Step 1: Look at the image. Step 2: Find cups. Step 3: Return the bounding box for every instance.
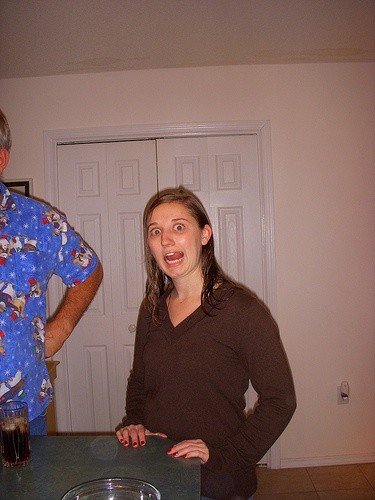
[0,401,32,468]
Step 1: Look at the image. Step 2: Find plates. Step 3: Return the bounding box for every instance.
[60,478,161,500]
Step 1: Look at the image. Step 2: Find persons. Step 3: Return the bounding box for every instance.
[0,110,103,435]
[115,187,297,500]
